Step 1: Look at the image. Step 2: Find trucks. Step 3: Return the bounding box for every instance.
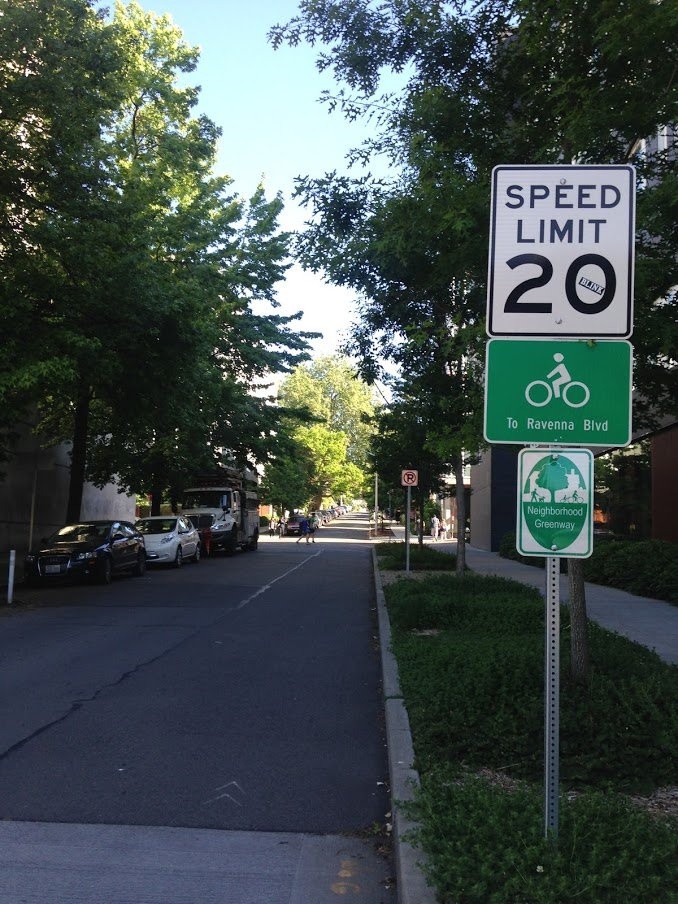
[179,475,260,556]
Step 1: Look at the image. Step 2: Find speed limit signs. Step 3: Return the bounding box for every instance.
[485,164,636,339]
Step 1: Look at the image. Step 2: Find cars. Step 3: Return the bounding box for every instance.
[135,514,202,568]
[285,504,354,536]
[25,519,146,586]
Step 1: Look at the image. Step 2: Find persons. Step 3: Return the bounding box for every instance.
[395,509,401,525]
[309,513,316,544]
[296,515,310,545]
[268,516,277,536]
[439,518,447,541]
[277,516,285,538]
[431,514,439,542]
